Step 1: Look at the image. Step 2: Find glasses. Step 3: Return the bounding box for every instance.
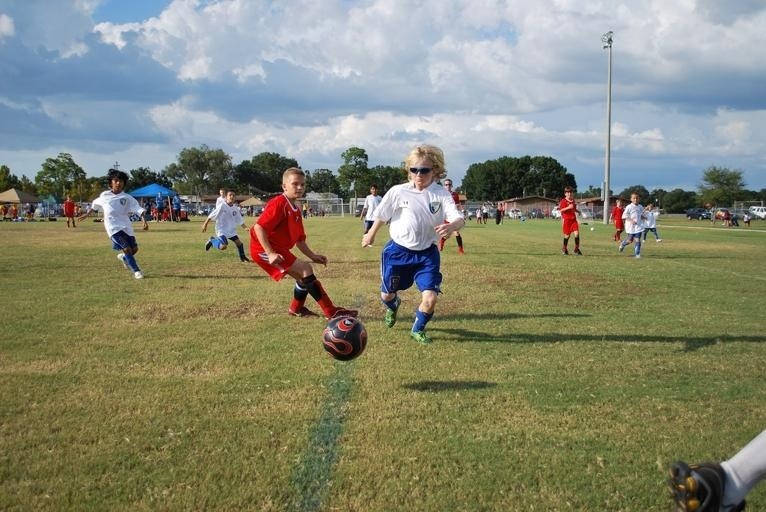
[409,167,434,174]
[444,183,452,186]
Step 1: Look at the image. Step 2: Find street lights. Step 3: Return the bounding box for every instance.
[600,31,615,225]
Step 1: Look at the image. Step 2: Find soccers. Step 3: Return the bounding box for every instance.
[322,317,368,362]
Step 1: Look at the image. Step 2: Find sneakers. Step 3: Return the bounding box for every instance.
[411,328,433,344]
[619,242,624,253]
[117,252,127,271]
[135,272,145,281]
[204,239,213,251]
[67,225,69,227]
[288,307,319,320]
[241,257,255,265]
[459,248,464,254]
[73,225,76,227]
[575,249,583,257]
[614,234,616,241]
[325,308,358,322]
[656,239,662,243]
[643,239,646,244]
[617,240,622,244]
[562,247,569,255]
[636,254,640,259]
[384,298,402,329]
[439,240,443,251]
[671,459,747,512]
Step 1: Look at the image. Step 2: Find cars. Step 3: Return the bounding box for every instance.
[528,208,544,218]
[687,208,711,220]
[356,205,364,216]
[715,208,732,220]
[729,210,756,223]
[509,209,522,218]
[469,207,477,216]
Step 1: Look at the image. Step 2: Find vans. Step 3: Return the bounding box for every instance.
[552,206,595,219]
[749,206,766,220]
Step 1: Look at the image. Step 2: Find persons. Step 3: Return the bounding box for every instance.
[156,192,164,223]
[144,198,158,221]
[64,195,77,228]
[463,200,551,225]
[202,190,253,263]
[78,169,149,280]
[189,207,215,216]
[643,204,662,243]
[74,202,91,218]
[172,193,182,222]
[609,199,626,242]
[237,206,267,217]
[0,202,63,224]
[438,178,464,255]
[710,208,754,229]
[667,426,765,512]
[560,186,583,256]
[216,188,226,209]
[363,145,465,343]
[360,184,383,235]
[302,201,331,219]
[619,192,649,258]
[249,168,359,322]
